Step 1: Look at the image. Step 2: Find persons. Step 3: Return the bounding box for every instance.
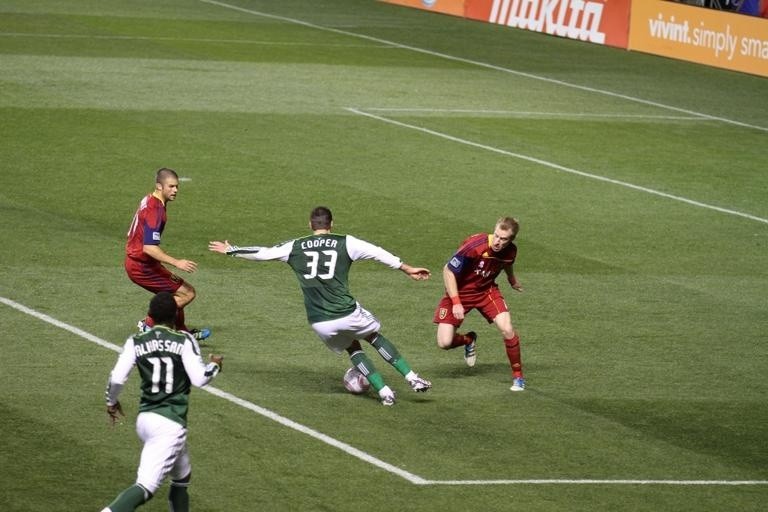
[100,292,223,512]
[433,216,528,391]
[124,167,210,340]
[208,205,431,406]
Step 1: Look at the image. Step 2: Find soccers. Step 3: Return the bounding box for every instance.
[342,365,370,393]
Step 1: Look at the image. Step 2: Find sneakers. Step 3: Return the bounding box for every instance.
[190,329,210,339]
[510,378,524,391]
[382,394,395,406]
[409,378,432,392]
[463,332,476,366]
[138,319,150,333]
